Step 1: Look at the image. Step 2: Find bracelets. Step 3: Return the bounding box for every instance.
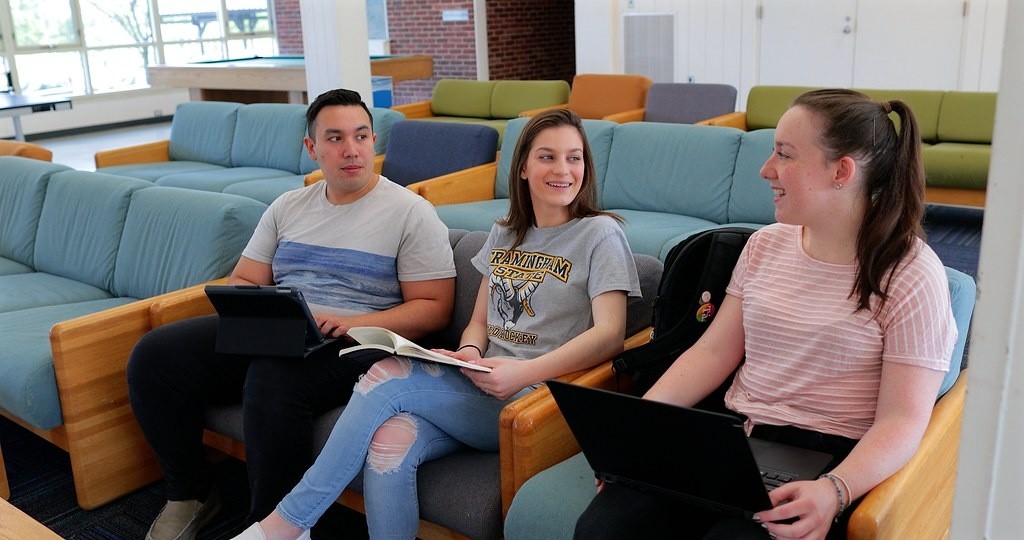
[457,344,481,356]
[819,472,852,523]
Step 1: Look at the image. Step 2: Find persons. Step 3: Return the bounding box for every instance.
[229,109,644,540]
[572,88,958,540]
[127,88,456,540]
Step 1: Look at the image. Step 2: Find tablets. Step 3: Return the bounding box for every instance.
[205,284,324,344]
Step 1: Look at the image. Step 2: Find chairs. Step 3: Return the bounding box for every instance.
[519,73,652,123]
[604,82,737,125]
[305,120,499,194]
[512,264,968,540]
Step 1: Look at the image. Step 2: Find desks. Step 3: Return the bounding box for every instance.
[146,54,432,104]
[0,91,72,142]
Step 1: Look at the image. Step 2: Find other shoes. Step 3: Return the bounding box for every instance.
[229,520,312,540]
[145,485,221,540]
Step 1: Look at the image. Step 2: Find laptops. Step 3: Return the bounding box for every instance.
[542,378,839,520]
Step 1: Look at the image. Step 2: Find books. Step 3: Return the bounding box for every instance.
[338,326,492,374]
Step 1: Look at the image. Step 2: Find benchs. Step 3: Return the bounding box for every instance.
[418,117,782,265]
[716,85,997,209]
[148,219,664,540]
[1,155,267,510]
[390,79,570,149]
[93,101,404,205]
